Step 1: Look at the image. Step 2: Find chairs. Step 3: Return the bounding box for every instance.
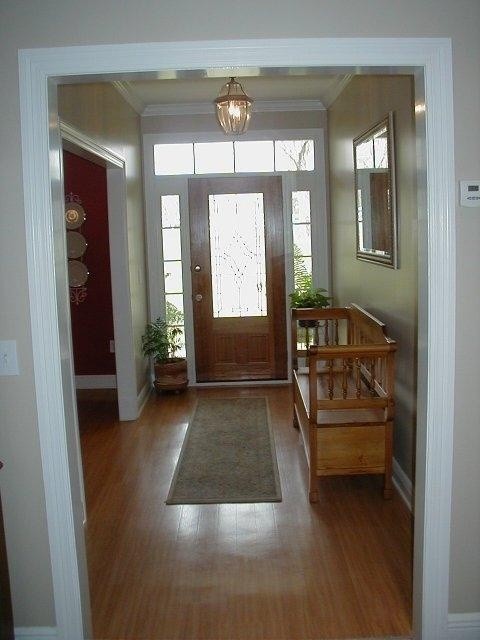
[289,302,398,503]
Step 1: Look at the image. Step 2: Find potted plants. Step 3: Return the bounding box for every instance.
[141,291,189,392]
[288,287,334,328]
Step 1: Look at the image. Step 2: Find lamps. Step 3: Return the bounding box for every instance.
[214,77,253,132]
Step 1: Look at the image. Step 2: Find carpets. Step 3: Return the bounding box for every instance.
[165,396,282,505]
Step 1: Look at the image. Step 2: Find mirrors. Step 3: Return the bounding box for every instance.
[353,111,398,269]
